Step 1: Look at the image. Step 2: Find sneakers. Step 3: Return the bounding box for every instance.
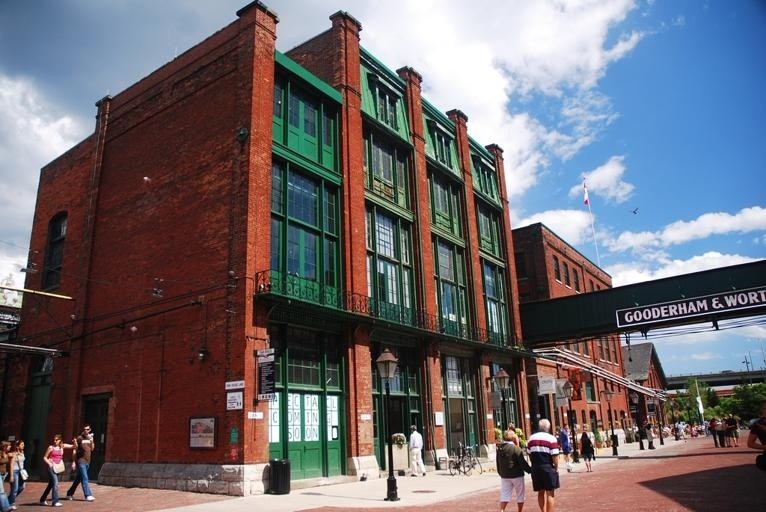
[67,495,74,501]
[40,499,49,506]
[2,505,18,512]
[85,496,96,502]
[54,502,63,507]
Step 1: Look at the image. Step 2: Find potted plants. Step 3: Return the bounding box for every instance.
[592,425,604,449]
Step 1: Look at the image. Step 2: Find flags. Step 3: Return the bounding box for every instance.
[583,182,590,205]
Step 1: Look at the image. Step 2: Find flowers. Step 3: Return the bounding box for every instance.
[391,432,407,446]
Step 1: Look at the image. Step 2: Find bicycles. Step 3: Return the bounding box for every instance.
[448,441,485,475]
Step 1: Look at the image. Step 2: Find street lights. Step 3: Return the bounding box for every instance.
[603,388,618,456]
[562,380,580,464]
[374,345,405,501]
[492,367,514,434]
[631,392,646,452]
[652,392,703,445]
[740,354,752,387]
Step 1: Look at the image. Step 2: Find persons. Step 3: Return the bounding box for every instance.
[408,425,426,476]
[494,400,766,512]
[0,422,96,512]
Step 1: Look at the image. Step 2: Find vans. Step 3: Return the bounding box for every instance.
[720,368,737,375]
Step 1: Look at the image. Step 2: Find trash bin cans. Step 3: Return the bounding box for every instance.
[270,459,291,494]
[626,430,634,443]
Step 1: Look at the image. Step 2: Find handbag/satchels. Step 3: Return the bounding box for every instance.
[52,460,66,474]
[20,469,29,481]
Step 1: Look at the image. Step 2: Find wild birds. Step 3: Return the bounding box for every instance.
[628,207,639,215]
